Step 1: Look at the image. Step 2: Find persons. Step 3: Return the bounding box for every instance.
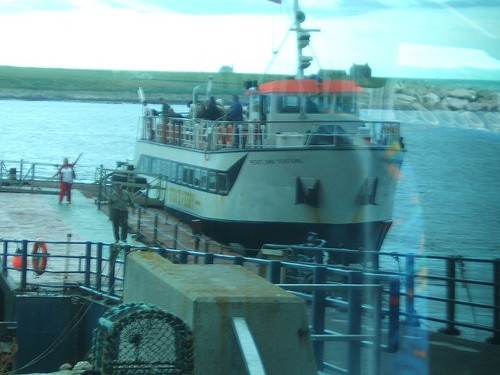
[142,100,152,138]
[195,98,221,139]
[217,94,244,144]
[109,181,135,244]
[166,108,185,125]
[50,151,84,206]
[156,97,170,123]
[306,97,318,113]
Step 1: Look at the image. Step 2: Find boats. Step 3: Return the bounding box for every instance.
[129,0,406,273]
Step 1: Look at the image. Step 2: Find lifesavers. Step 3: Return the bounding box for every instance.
[225,126,233,145]
[32,238,48,275]
[217,126,223,143]
